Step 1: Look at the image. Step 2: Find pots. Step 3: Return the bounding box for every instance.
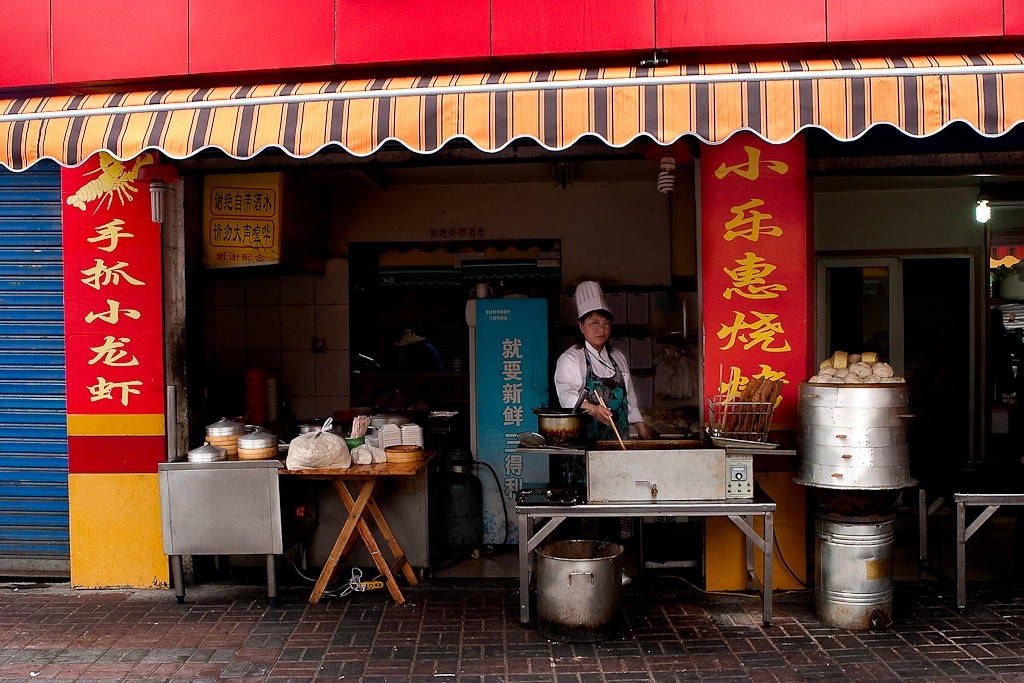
[532,408,590,445]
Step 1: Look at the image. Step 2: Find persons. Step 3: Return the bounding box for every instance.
[554,282,697,586]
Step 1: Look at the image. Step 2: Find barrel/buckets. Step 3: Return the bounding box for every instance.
[534,540,625,643]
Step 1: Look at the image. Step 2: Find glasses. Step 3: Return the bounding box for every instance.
[581,321,610,329]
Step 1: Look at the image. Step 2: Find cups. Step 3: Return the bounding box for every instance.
[475,283,493,298]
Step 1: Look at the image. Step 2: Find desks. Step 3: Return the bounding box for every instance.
[914,478,1024,613]
[278,452,436,605]
[512,483,777,630]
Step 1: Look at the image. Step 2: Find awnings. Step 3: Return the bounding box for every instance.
[0,50,1024,173]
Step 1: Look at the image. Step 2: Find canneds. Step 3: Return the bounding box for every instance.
[476,284,488,299]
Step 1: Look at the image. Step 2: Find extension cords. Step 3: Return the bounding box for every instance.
[359,581,384,589]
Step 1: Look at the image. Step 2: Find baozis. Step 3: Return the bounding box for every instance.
[807,354,906,383]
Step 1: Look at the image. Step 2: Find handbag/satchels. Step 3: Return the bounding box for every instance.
[286,417,352,473]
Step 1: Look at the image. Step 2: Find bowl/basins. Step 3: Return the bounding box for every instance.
[378,423,424,451]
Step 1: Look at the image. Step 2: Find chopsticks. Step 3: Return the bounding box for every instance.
[594,389,626,451]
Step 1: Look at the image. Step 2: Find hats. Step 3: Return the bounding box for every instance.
[576,280,615,319]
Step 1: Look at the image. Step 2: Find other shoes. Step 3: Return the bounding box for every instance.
[621,573,631,585]
